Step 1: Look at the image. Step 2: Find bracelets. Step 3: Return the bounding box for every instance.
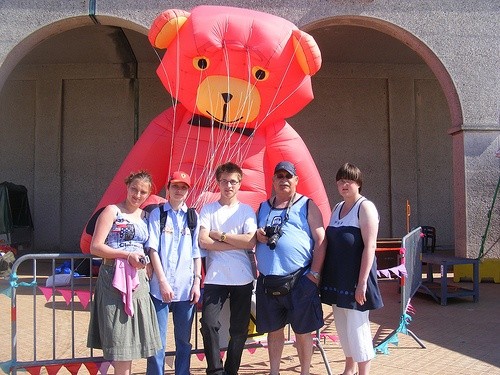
[128,252,132,260]
[194,274,202,279]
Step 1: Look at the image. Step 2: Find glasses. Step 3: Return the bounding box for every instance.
[218,179,239,185]
[274,173,294,179]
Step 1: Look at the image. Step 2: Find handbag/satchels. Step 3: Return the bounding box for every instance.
[257,263,312,296]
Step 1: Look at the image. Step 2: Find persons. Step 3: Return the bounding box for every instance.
[86,170,153,375]
[319,163,384,375]
[145,170,207,375]
[198,162,257,375]
[256,161,327,375]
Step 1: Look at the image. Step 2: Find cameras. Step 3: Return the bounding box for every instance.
[264,226,283,250]
[138,255,151,265]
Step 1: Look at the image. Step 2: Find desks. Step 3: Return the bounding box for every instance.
[416,253,479,306]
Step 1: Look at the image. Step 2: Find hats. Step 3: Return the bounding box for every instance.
[169,172,192,189]
[275,161,296,175]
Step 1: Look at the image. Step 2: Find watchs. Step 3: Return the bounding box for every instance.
[309,269,321,280]
[219,232,227,243]
